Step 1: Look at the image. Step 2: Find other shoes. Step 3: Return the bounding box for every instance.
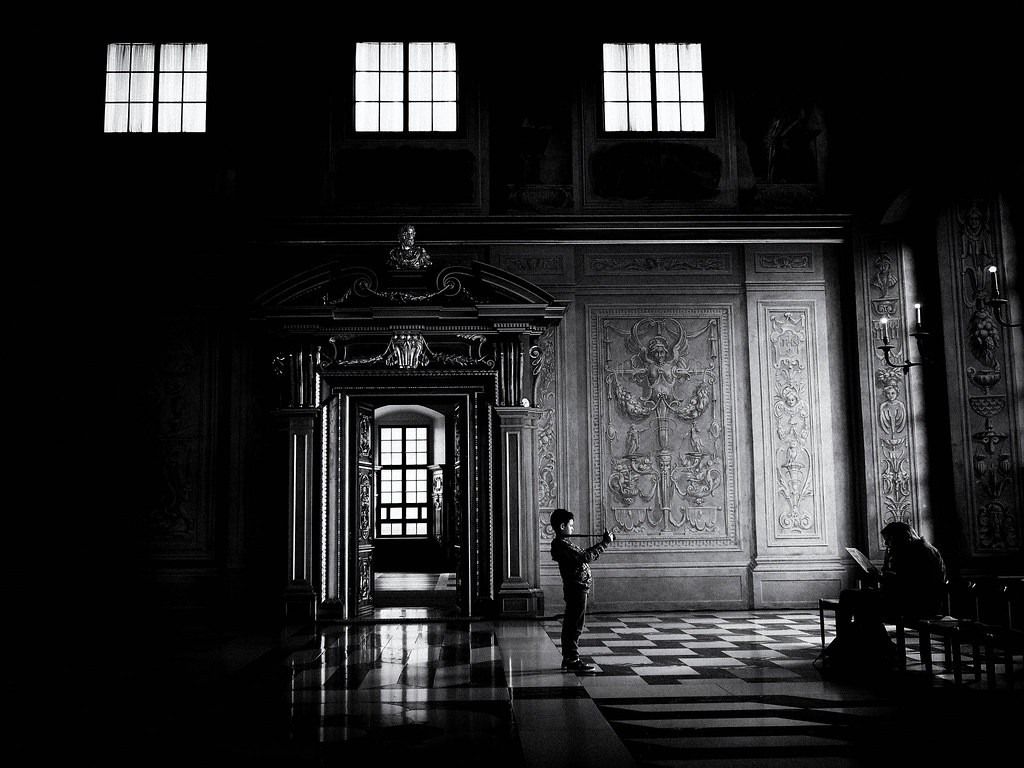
[561,658,595,671]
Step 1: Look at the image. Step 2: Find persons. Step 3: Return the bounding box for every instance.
[550,509,614,672]
[647,336,676,398]
[879,381,906,434]
[836,522,946,672]
[773,385,810,446]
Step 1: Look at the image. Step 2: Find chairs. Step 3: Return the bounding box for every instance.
[816,574,1024,694]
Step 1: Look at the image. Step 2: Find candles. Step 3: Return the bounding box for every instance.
[918,303,921,323]
[991,267,998,290]
[883,318,888,337]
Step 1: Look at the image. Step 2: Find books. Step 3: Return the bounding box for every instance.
[844,547,881,575]
